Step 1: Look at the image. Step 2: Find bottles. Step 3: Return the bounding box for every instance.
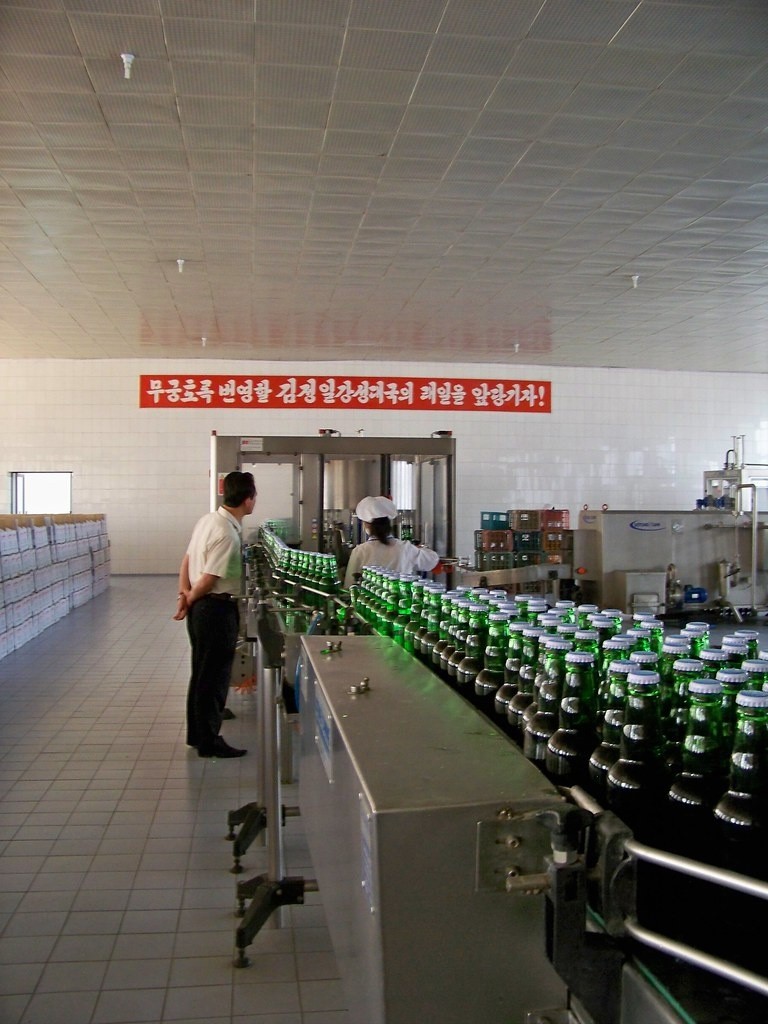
[258,520,768,1024]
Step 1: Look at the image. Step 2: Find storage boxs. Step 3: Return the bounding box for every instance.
[474,509,570,593]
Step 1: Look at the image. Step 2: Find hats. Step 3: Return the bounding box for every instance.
[356,496,398,523]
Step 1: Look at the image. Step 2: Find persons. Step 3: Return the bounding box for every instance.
[172,471,247,757]
[344,496,438,588]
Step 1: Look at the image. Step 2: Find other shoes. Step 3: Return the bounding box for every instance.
[222,708,236,720]
[198,735,247,758]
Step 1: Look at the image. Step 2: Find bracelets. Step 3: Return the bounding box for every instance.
[177,596,180,600]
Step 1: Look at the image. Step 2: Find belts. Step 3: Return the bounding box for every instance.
[209,594,237,603]
